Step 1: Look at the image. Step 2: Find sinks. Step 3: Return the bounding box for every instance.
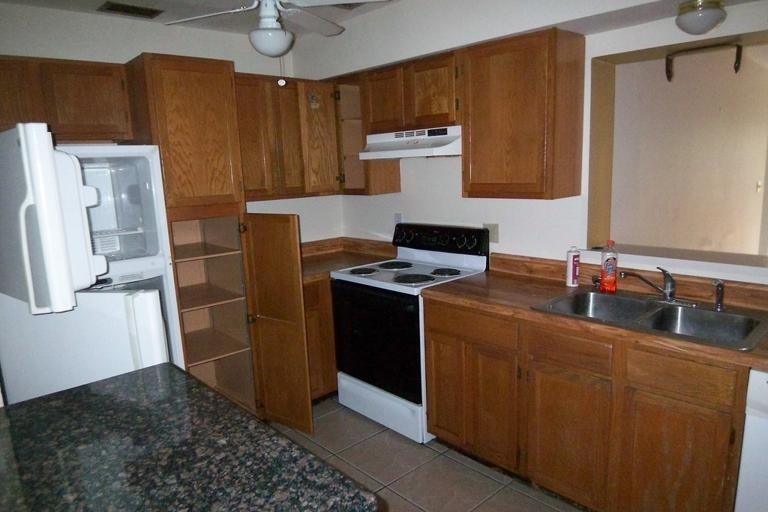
[531,287,663,330]
[630,298,768,353]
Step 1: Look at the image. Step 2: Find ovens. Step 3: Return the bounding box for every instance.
[328,278,437,445]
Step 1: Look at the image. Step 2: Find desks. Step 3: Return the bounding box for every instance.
[0,362,389,512]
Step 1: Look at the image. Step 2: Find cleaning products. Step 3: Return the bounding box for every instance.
[600,239,618,291]
[565,246,580,288]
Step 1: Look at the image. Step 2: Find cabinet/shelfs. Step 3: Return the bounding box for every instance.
[525,315,622,512]
[297,70,402,195]
[235,71,318,202]
[167,202,314,435]
[423,295,520,475]
[300,276,338,402]
[613,332,750,512]
[125,52,242,208]
[363,49,462,137]
[463,27,585,199]
[0,54,134,144]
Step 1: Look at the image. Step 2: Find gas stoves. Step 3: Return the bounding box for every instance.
[328,220,491,295]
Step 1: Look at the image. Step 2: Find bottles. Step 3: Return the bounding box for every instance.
[600,241,616,293]
[567,248,581,288]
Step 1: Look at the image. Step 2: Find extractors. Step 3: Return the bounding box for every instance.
[355,124,461,161]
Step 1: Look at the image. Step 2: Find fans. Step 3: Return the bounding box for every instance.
[165,0,390,37]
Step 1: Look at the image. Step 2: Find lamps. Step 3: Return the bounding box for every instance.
[249,0,294,57]
[675,0,727,35]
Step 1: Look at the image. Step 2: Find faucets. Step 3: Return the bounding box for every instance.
[711,279,726,312]
[619,266,676,298]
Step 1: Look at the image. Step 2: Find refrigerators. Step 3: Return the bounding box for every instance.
[0,121,186,407]
